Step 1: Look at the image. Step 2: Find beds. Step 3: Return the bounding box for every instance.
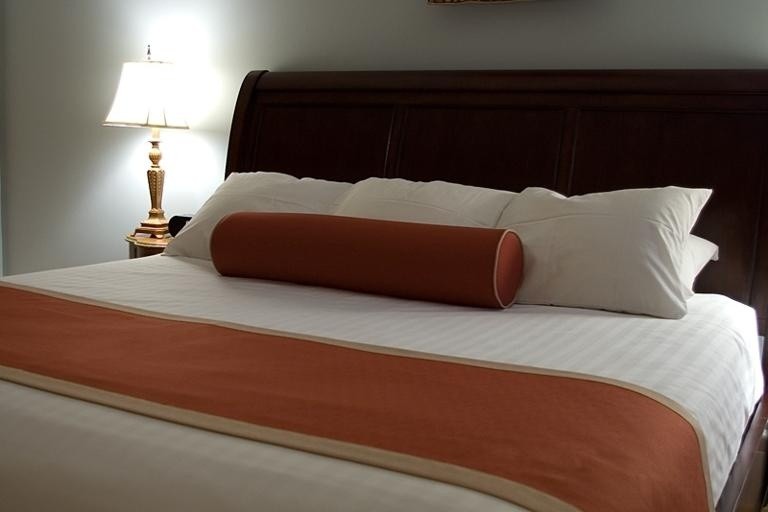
[1,69,768,511]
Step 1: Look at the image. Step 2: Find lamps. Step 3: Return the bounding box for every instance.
[99,43,192,239]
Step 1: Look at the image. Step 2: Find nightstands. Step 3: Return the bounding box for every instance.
[122,233,173,259]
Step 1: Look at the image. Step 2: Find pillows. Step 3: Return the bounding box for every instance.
[493,184,713,319]
[685,229,723,299]
[208,212,527,313]
[165,172,351,262]
[337,175,520,232]
